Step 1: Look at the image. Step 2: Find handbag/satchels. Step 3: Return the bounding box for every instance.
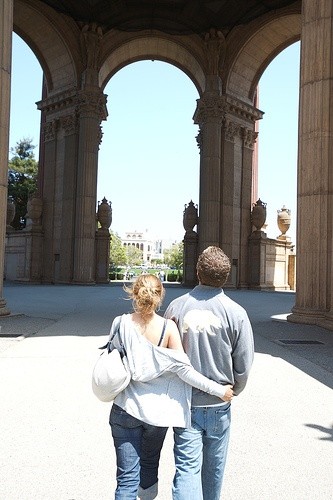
[92,315,131,402]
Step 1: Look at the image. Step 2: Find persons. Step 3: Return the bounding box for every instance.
[162,245,254,500]
[109,272,234,500]
